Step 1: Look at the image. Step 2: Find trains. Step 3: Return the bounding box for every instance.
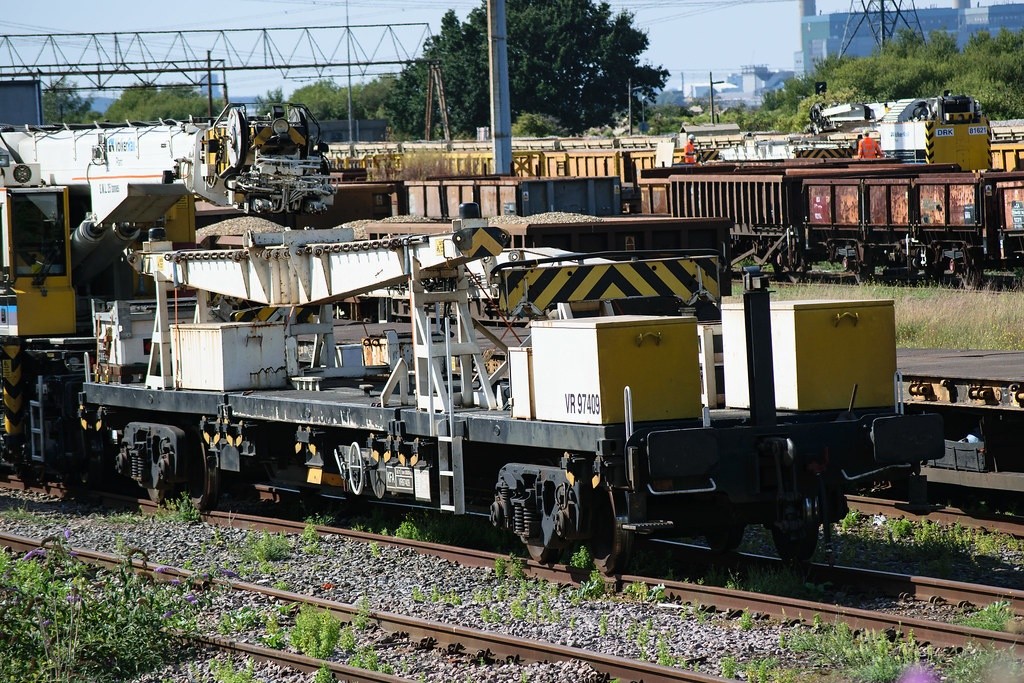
[181,100,1023,290]
[0,146,945,576]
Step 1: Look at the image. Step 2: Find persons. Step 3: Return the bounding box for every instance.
[858,129,883,158]
[683,133,697,164]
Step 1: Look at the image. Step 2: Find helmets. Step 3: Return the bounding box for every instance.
[862,129,870,135]
[688,134,695,140]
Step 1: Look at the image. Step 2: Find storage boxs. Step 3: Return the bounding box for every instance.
[170,321,288,391]
[507,298,897,427]
[99,319,196,368]
